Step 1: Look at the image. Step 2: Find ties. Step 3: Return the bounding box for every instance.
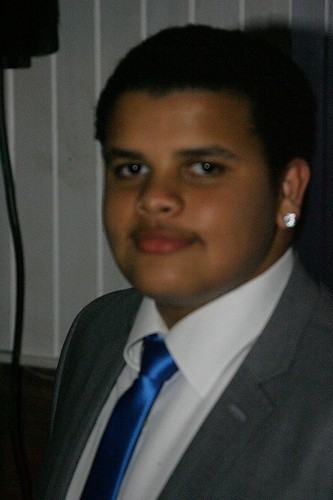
[75,332,180,500]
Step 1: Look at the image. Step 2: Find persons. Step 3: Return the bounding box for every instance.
[38,21,333,499]
